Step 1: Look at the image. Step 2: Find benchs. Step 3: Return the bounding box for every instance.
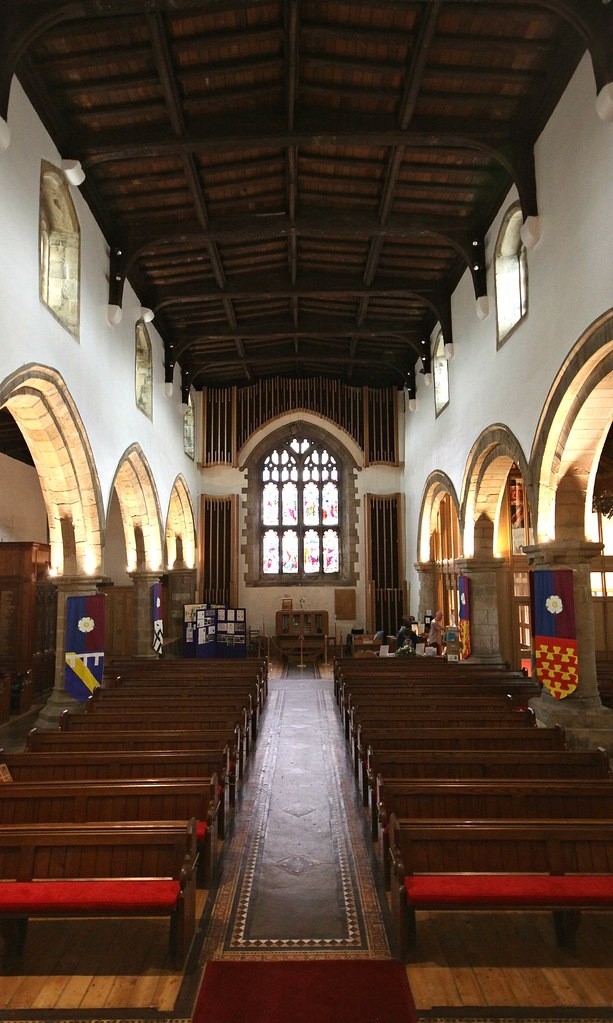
[332,655,613,972]
[0,655,268,967]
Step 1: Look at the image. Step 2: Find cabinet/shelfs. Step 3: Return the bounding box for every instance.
[351,634,383,656]
[275,609,328,664]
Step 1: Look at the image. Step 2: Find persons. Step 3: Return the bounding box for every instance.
[429,611,446,655]
[397,620,429,649]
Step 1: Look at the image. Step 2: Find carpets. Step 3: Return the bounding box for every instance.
[191,958,418,1023]
